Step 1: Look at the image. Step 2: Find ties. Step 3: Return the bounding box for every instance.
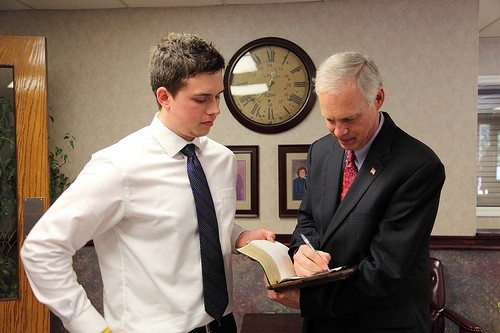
[180,143,230,320]
[340,149,358,203]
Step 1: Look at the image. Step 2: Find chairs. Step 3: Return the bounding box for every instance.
[428,258,482,333]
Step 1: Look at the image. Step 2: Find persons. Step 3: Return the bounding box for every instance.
[292,166,307,200]
[264,51,447,333]
[19,33,275,333]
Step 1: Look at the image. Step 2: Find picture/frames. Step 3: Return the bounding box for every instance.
[278,144,311,218]
[224,145,260,218]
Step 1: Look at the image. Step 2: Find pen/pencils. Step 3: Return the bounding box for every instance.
[302,233,330,271]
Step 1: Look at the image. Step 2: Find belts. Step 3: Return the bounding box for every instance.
[189,312,235,333]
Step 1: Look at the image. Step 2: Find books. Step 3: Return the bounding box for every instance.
[236,239,354,293]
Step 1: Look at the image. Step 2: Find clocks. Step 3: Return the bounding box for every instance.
[223,36,317,134]
